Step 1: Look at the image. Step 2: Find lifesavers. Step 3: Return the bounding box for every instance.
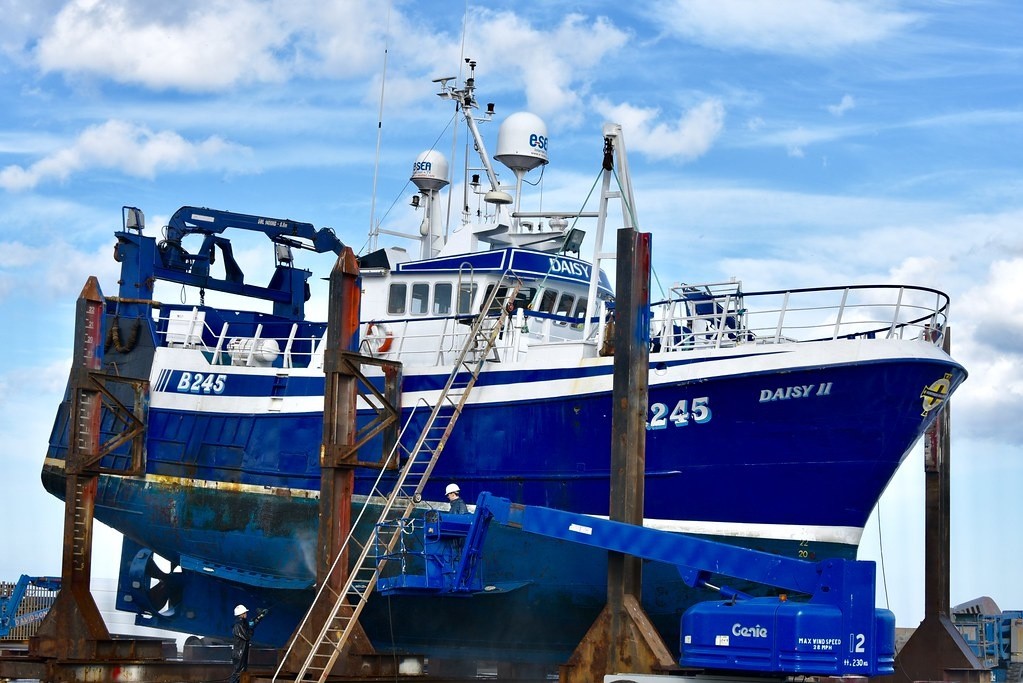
[363,320,393,354]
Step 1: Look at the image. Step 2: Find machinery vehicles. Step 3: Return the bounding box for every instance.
[374,488,898,683]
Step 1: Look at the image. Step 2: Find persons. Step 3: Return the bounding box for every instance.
[228,604,268,683]
[444,482,468,515]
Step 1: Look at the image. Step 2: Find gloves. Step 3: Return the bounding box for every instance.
[248,621,254,629]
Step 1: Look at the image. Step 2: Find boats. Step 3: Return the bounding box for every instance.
[40,27,968,666]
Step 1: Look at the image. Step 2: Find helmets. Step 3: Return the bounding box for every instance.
[445,483,460,495]
[234,604,249,616]
